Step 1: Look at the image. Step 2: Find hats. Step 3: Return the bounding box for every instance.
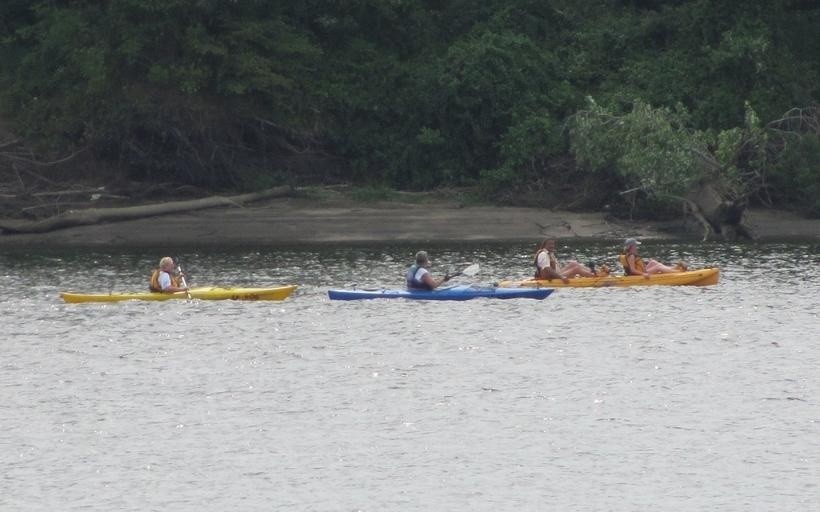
[624,238,642,247]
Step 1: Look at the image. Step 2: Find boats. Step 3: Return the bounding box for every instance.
[327,287,555,302]
[57,284,299,307]
[499,268,722,293]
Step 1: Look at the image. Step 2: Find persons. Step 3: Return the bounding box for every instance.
[149,256,189,292]
[171,256,178,288]
[403,250,448,291]
[424,257,433,269]
[534,237,610,284]
[621,234,689,279]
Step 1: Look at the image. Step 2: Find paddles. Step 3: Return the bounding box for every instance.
[448,264,479,278]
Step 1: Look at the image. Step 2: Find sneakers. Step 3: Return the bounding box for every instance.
[676,261,687,271]
[599,264,608,276]
